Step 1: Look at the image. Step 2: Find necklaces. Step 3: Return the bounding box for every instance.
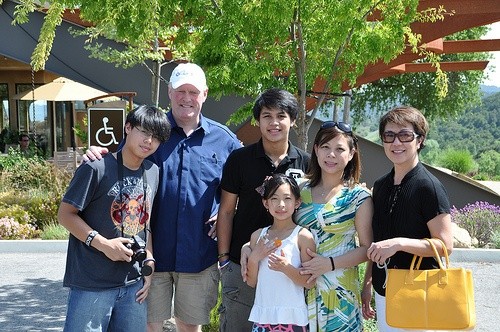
[318,184,332,196]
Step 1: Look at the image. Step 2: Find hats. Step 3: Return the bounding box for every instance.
[170,62,206,91]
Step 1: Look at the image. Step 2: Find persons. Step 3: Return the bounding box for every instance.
[248,172,317,332]
[18,134,34,158]
[79,62,243,332]
[239,120,374,332]
[55,104,171,332]
[361,105,454,332]
[216,89,313,331]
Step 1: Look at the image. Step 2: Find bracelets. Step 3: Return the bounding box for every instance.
[142,258,156,267]
[328,257,335,272]
[84,230,99,247]
[217,252,230,260]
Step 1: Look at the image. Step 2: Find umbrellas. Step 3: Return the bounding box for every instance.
[12,77,122,171]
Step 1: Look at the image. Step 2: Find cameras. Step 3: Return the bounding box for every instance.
[122,235,147,262]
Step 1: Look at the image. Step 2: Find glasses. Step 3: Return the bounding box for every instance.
[381,130,420,144]
[132,123,163,143]
[320,121,353,137]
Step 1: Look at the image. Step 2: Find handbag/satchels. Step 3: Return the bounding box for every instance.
[385,237,476,331]
